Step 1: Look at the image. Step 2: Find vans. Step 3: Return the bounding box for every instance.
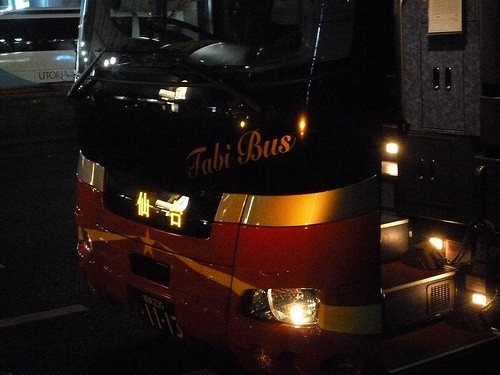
[2,6,217,154]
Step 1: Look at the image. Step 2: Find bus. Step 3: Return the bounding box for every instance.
[71,1,500,374]
